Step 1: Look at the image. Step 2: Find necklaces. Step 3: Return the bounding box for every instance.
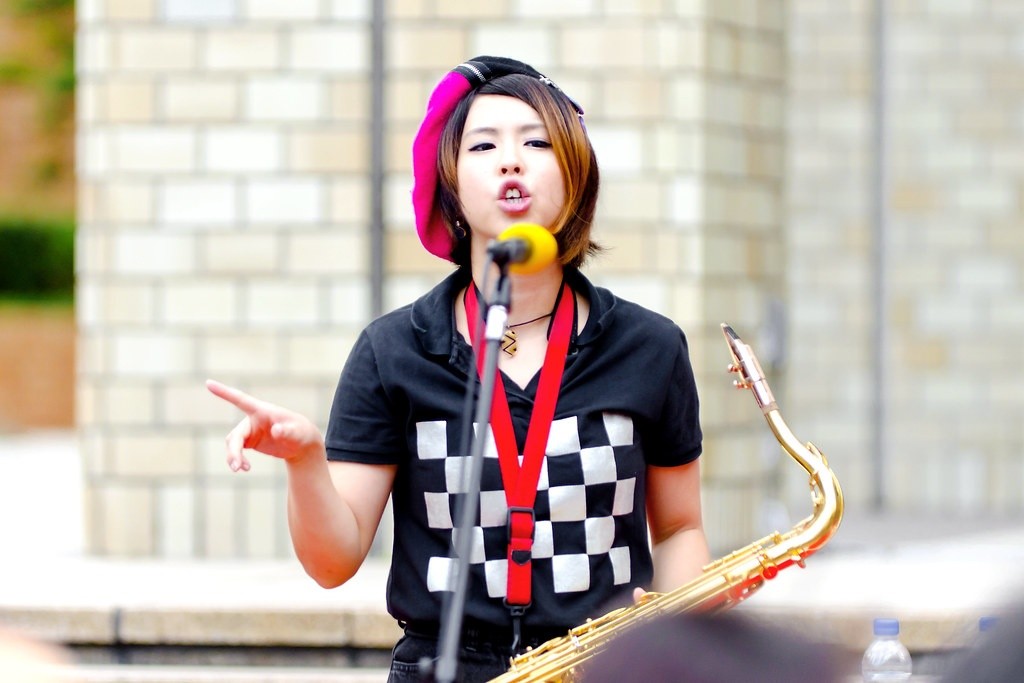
[464,282,559,358]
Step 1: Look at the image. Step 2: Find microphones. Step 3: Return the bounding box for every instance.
[487,222,558,274]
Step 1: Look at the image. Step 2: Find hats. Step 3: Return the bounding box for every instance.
[410,54,588,267]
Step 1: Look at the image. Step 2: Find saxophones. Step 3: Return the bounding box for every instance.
[480,321,846,683]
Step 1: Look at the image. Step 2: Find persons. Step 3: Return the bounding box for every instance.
[204,56,728,683]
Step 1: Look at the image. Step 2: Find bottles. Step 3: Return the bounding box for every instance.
[861,619,913,683]
